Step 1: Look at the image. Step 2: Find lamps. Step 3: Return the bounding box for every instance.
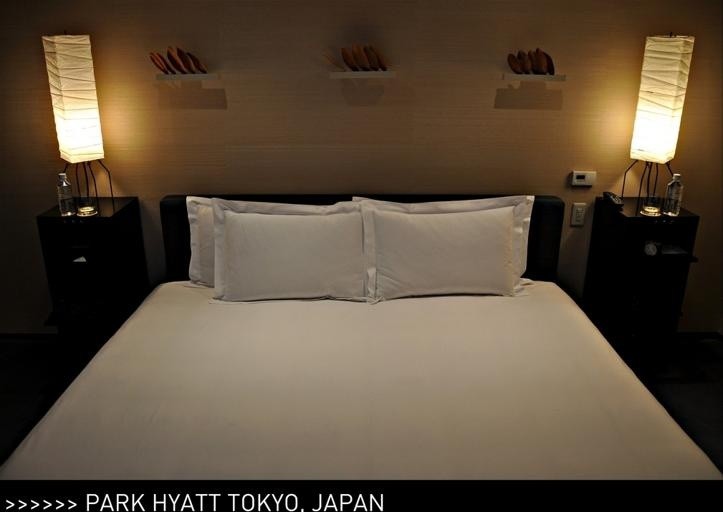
[38,33,115,215]
[622,36,696,212]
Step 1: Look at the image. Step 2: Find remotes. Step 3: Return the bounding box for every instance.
[603,192,624,207]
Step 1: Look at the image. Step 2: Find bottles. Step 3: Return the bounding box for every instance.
[662,173,684,217]
[55,172,76,217]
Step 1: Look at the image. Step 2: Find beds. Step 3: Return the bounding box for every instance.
[2,194,723,482]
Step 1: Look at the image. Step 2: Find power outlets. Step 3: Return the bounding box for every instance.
[571,201,586,227]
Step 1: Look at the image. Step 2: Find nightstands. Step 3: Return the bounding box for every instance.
[583,194,700,369]
[35,196,152,327]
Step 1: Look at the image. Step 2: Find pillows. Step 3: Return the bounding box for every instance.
[185,195,536,300]
